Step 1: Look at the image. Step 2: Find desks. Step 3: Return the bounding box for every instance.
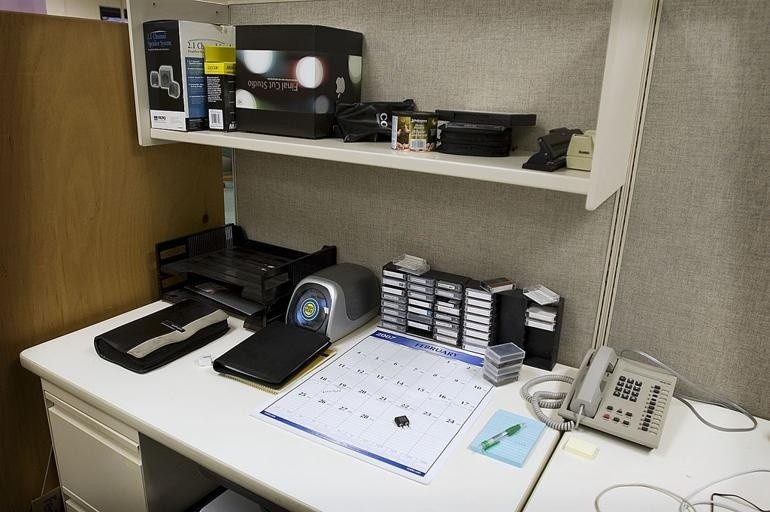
[20,299,580,512]
[522,399,770,512]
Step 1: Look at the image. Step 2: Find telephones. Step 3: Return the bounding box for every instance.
[556,347,675,449]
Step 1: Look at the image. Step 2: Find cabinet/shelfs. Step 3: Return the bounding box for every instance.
[125,1,660,212]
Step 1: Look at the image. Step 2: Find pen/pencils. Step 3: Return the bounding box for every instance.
[477,424,527,452]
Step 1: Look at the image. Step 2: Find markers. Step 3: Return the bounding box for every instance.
[213,321,331,390]
